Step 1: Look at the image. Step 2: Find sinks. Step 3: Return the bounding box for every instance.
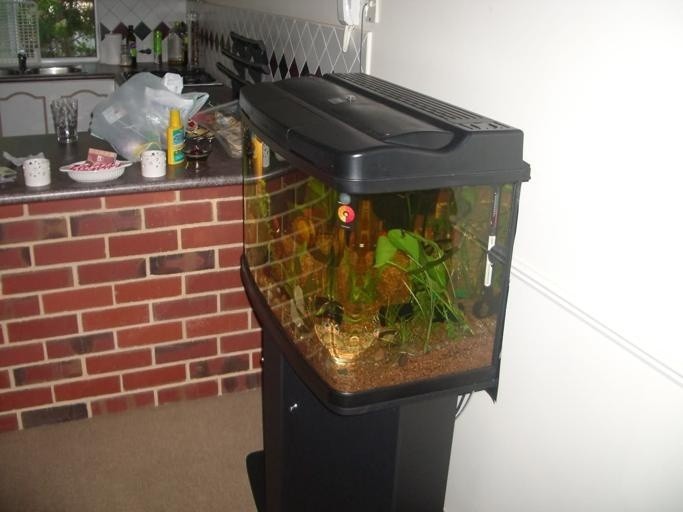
[119,65,223,87]
[0,64,86,80]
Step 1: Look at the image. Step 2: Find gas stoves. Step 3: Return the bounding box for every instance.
[123,70,216,84]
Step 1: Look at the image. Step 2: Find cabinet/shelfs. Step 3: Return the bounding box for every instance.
[245,329,457,511]
[0,79,120,138]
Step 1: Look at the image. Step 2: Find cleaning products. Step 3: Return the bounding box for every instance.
[166,109,185,165]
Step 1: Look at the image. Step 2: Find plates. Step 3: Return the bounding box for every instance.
[58,159,134,185]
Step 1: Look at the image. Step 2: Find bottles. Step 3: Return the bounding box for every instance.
[125,25,138,68]
[154,31,161,64]
[167,107,184,165]
[169,22,188,64]
[18,50,27,76]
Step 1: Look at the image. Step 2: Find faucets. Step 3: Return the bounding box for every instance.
[18,50,28,69]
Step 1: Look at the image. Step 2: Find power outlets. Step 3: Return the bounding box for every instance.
[361,0,378,23]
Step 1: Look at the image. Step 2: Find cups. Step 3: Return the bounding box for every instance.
[22,158,51,187]
[50,97,80,146]
[140,150,167,178]
[183,138,215,172]
